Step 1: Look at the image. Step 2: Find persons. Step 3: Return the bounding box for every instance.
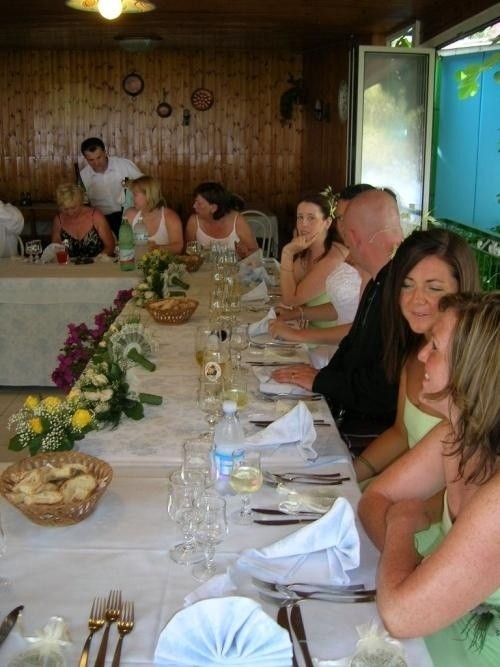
[354,290,500,667]
[183,180,258,264]
[75,137,145,261]
[269,188,407,455]
[48,181,114,261]
[0,194,26,258]
[352,226,481,496]
[278,193,348,350]
[122,174,184,259]
[267,184,375,372]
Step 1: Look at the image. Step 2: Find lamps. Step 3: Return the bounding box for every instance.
[65,0,156,21]
[113,33,163,53]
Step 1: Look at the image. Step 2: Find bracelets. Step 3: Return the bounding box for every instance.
[298,306,305,321]
[279,265,294,273]
[356,455,376,476]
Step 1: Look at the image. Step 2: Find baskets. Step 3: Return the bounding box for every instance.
[146,297,199,324]
[0,452,113,526]
[180,256,202,272]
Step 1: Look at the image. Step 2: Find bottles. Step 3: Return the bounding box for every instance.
[74,162,89,205]
[211,401,246,495]
[119,215,134,271]
[133,217,150,277]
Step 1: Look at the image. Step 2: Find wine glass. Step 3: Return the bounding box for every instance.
[166,469,207,566]
[195,240,249,423]
[186,241,195,264]
[181,439,219,512]
[32,240,42,264]
[229,447,263,524]
[190,496,231,580]
[25,241,35,264]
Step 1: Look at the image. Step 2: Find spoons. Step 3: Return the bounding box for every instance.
[272,474,351,481]
[276,582,377,600]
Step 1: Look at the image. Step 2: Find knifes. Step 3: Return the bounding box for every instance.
[290,605,313,666]
[0,605,23,643]
[250,507,326,516]
[277,605,300,666]
[256,423,331,428]
[251,420,325,424]
[254,518,317,525]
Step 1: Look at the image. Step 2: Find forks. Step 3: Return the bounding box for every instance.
[256,393,322,401]
[78,598,106,666]
[93,590,122,666]
[260,469,340,480]
[111,601,135,666]
[252,577,365,598]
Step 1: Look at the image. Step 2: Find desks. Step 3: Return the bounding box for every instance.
[15,198,60,241]
[239,213,279,259]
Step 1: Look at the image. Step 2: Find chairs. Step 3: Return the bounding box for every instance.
[17,235,25,256]
[240,210,273,261]
[110,230,118,256]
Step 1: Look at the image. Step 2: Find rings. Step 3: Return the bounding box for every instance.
[290,371,296,379]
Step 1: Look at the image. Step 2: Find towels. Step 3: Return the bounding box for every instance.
[150,593,297,667]
[234,492,363,591]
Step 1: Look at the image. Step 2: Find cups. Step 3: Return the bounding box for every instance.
[55,245,67,264]
